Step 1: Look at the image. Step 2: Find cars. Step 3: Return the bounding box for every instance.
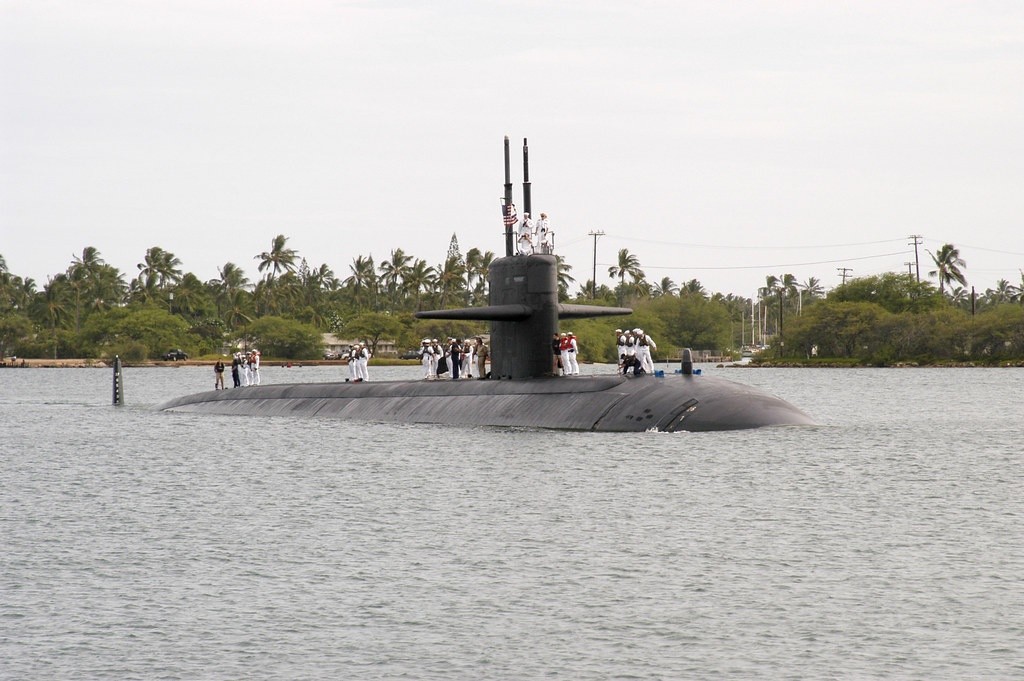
[322,349,375,361]
[398,350,423,360]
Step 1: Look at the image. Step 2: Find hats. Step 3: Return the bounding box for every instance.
[349,343,364,349]
[422,337,470,345]
[560,333,566,336]
[637,330,644,335]
[567,332,573,335]
[620,354,625,359]
[623,330,630,334]
[615,329,622,332]
[633,328,641,332]
[233,349,257,359]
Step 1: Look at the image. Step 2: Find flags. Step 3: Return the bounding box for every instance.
[502,204,518,225]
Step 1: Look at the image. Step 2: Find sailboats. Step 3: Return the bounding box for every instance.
[740,291,771,357]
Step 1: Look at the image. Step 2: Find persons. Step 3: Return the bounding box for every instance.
[349,343,369,382]
[419,337,488,380]
[535,213,549,253]
[616,328,657,375]
[517,213,534,256]
[552,332,579,376]
[232,350,260,388]
[214,358,225,390]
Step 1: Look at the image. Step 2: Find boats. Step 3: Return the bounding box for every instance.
[105,131,825,437]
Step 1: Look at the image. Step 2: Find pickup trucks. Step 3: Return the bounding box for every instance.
[161,347,189,362]
